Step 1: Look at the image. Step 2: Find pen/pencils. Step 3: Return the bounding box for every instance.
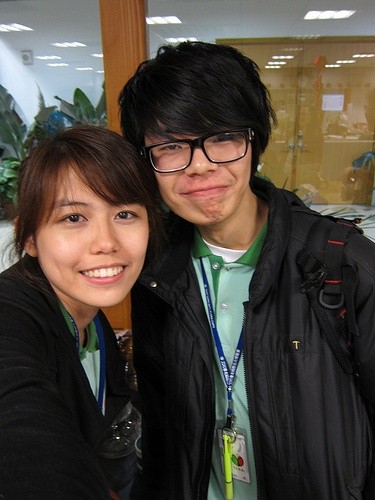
[221,435,234,500]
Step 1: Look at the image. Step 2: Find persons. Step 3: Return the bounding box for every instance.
[117,40,375,500]
[1,125,186,500]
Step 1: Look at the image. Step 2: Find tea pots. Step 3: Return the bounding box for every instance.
[101,400,142,458]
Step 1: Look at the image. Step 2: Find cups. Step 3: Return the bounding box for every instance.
[135,436,143,473]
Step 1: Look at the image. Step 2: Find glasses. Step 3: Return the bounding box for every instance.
[140,128,255,173]
